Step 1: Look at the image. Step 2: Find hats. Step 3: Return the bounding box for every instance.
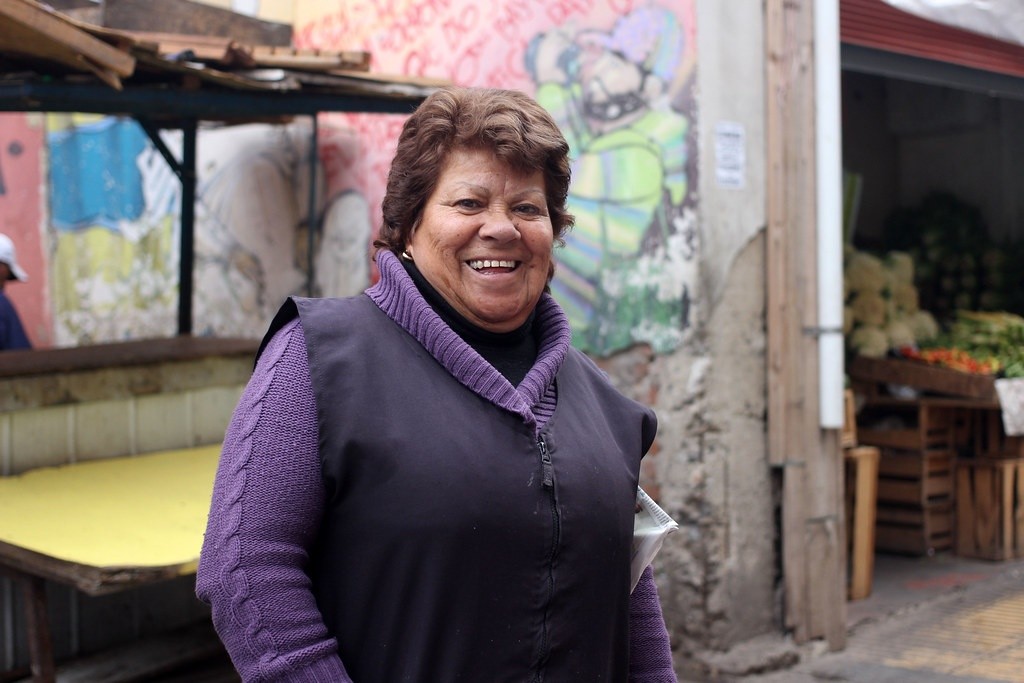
[1,233,30,282]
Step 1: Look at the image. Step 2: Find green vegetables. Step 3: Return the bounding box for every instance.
[916,313,1024,376]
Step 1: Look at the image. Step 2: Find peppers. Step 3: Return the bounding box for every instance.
[897,342,998,376]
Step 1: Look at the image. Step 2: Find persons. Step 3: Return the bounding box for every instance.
[0,232,36,353]
[190,85,681,682]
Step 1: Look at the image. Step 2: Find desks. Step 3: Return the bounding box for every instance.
[849,393,1003,557]
[0,445,224,683]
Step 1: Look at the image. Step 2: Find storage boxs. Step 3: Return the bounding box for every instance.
[955,454,1024,563]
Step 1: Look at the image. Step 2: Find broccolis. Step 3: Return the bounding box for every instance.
[842,248,938,359]
